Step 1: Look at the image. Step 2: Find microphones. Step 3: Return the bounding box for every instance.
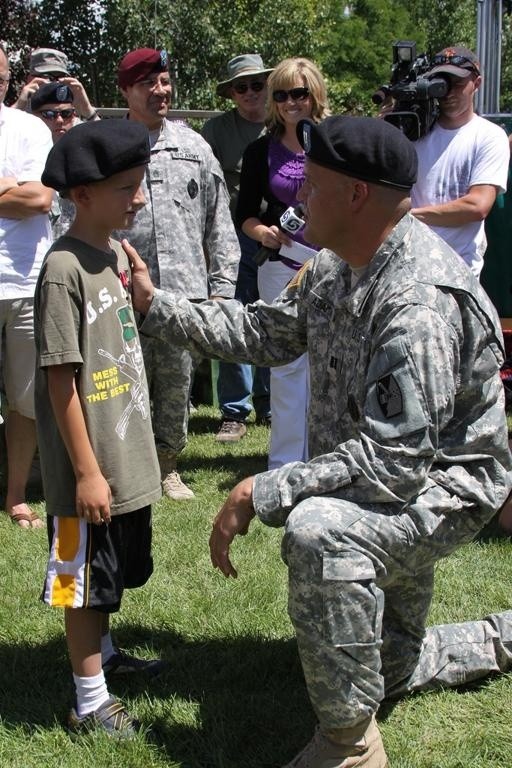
[252,203,304,266]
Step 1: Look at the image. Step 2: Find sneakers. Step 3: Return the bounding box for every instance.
[214,419,247,441]
[66,692,161,746]
[101,648,172,683]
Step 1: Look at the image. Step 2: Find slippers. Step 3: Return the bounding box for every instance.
[10,511,40,529]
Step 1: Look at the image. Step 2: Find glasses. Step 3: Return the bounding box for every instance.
[272,87,310,102]
[34,107,75,120]
[431,54,481,75]
[232,81,266,94]
[30,71,67,82]
[0,77,10,84]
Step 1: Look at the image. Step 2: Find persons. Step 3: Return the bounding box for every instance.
[34,115,162,738]
[0,45,512,531]
[122,118,512,768]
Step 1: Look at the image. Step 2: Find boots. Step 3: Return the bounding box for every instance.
[159,455,195,500]
[285,711,388,767]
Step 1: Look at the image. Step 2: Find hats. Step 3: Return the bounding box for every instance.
[29,47,71,76]
[30,82,73,109]
[118,47,169,86]
[216,53,275,99]
[41,118,151,191]
[427,46,481,79]
[295,113,418,190]
